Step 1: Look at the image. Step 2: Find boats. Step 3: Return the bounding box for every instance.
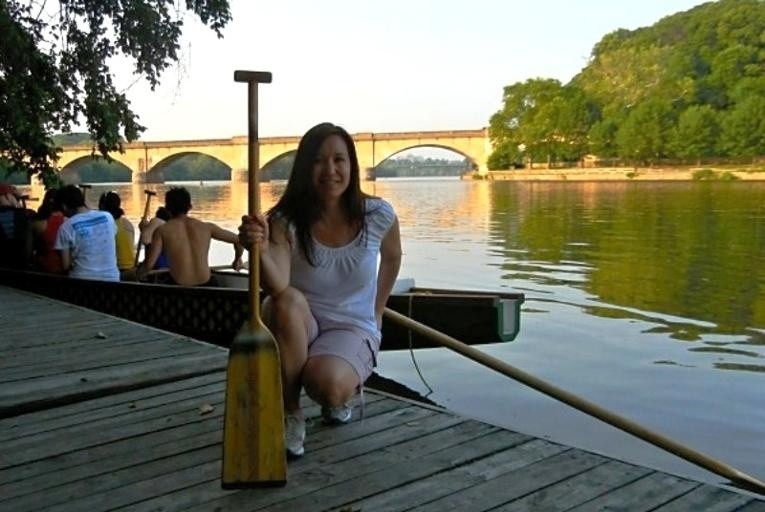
[4,238,525,354]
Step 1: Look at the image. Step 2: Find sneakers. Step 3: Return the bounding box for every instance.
[321,403,353,424]
[283,405,315,458]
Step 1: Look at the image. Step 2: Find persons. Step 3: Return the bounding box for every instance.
[0,174,136,283]
[237,121,402,459]
[138,206,171,275]
[126,186,244,288]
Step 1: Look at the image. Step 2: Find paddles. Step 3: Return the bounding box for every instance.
[225,69,287,490]
